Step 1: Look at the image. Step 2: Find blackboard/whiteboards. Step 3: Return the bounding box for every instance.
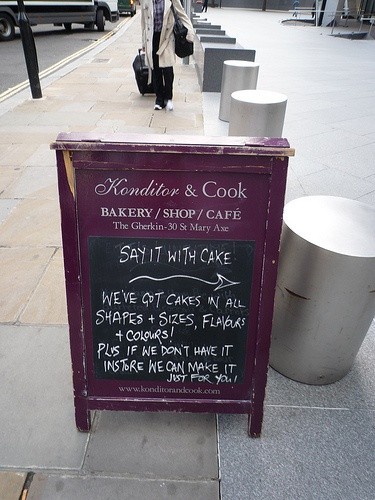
[53,128,297,413]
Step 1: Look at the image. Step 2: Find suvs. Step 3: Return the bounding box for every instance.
[0,0,122,42]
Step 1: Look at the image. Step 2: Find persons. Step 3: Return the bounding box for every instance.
[201,0,209,12]
[311,3,316,17]
[292,0,299,17]
[141,0,195,111]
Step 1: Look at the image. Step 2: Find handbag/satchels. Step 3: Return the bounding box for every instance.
[168,0,193,58]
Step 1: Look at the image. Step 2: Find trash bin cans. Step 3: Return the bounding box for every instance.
[193,1,203,13]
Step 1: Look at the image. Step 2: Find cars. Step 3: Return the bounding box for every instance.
[119,0,138,16]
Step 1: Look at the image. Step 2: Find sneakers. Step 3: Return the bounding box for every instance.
[166,100,173,110]
[153,104,163,110]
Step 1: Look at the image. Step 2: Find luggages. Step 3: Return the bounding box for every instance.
[133,49,156,95]
